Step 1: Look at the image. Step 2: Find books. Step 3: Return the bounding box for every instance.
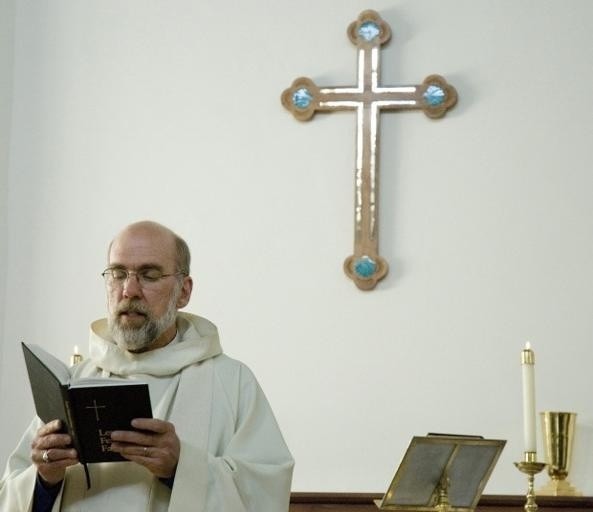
[19,339,156,464]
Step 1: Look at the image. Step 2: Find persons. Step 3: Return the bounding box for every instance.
[0,220,295,512]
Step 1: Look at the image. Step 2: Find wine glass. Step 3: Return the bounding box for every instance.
[537,408,585,496]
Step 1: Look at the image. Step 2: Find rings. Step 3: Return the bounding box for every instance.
[144,445,150,456]
[42,449,50,464]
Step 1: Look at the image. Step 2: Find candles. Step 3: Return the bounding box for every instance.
[519,338,538,462]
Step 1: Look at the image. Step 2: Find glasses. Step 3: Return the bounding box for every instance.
[100,267,181,285]
